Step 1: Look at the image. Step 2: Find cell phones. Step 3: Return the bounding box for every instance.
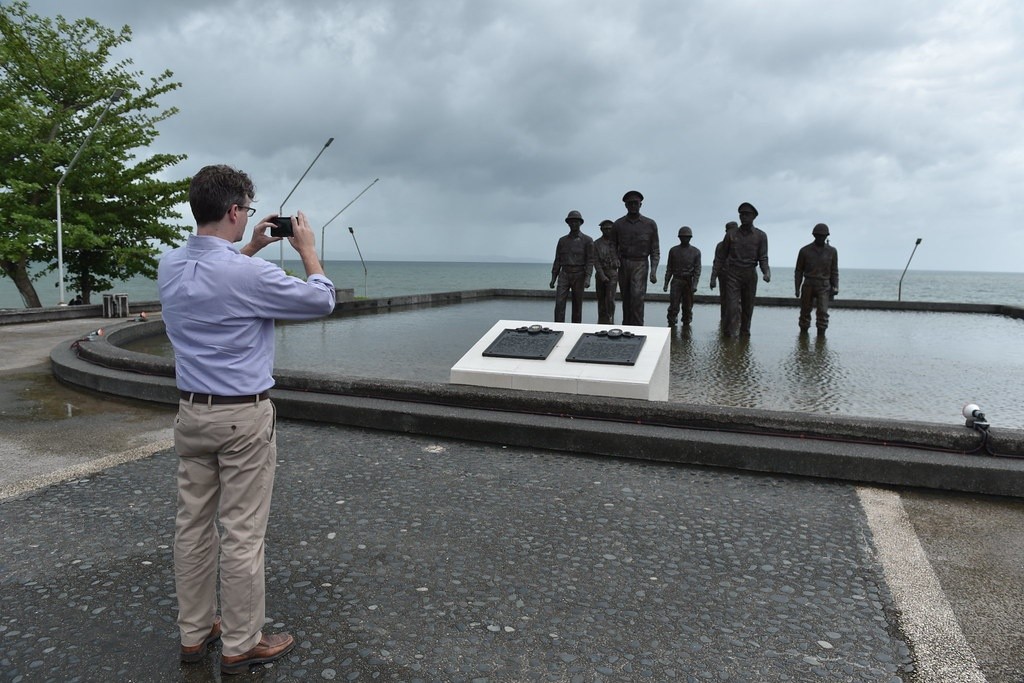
[271,216,299,238]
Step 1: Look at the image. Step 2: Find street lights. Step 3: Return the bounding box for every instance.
[348,227,366,269]
[321,177,379,268]
[899,237,922,300]
[56,87,124,306]
[280,137,335,267]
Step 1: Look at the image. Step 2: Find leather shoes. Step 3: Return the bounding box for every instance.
[220,632,296,674]
[181,615,221,662]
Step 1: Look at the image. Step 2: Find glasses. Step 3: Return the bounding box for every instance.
[228,204,256,217]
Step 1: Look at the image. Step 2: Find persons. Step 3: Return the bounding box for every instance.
[73,295,84,304]
[662,226,702,326]
[157,165,336,673]
[710,202,771,335]
[714,222,738,320]
[549,211,595,323]
[794,223,839,339]
[594,220,619,324]
[610,191,660,326]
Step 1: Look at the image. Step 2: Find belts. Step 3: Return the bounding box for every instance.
[178,390,269,404]
